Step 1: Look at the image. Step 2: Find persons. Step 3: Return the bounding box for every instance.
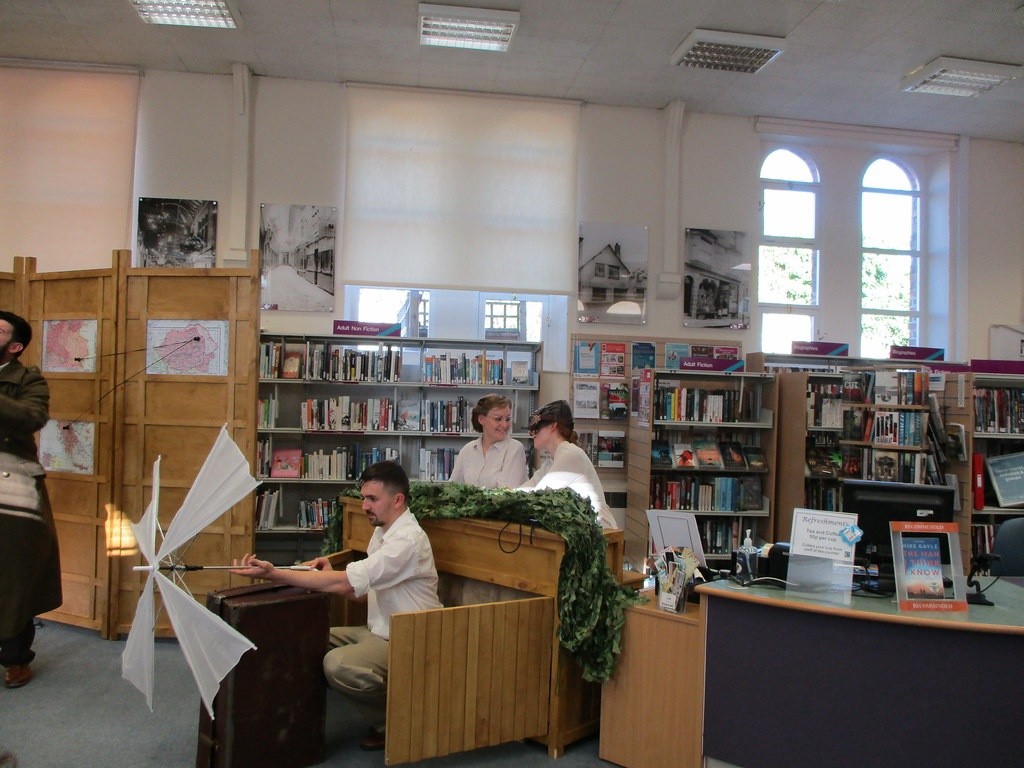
[226,461,441,749]
[514,400,619,532]
[447,393,529,489]
[0,311,62,687]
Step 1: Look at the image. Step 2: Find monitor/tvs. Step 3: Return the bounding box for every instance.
[843,479,958,593]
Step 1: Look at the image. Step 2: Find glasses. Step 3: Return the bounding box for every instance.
[486,416,513,423]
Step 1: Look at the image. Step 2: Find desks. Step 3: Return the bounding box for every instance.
[595,588,705,768]
[698,567,1024,768]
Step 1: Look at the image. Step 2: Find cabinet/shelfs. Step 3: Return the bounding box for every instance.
[945,372,1024,576]
[297,502,647,768]
[625,369,780,575]
[779,365,930,542]
[254,334,543,535]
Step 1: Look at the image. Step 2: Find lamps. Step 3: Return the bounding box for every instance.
[417,3,521,52]
[128,0,244,29]
[966,554,1002,606]
[669,28,788,75]
[899,56,1024,97]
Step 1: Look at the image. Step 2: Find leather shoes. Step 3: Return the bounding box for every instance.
[360,726,386,751]
[4,664,32,688]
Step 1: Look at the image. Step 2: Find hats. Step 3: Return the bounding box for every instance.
[520,400,573,429]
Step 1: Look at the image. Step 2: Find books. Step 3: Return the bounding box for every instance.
[972,386,1024,433]
[767,363,932,483]
[420,448,459,482]
[422,398,475,433]
[970,524,999,557]
[297,496,337,528]
[423,353,503,387]
[805,478,844,513]
[299,396,393,430]
[654,385,763,426]
[652,428,768,472]
[256,394,277,430]
[254,488,279,530]
[700,517,737,553]
[649,474,763,513]
[258,339,400,384]
[255,438,397,481]
